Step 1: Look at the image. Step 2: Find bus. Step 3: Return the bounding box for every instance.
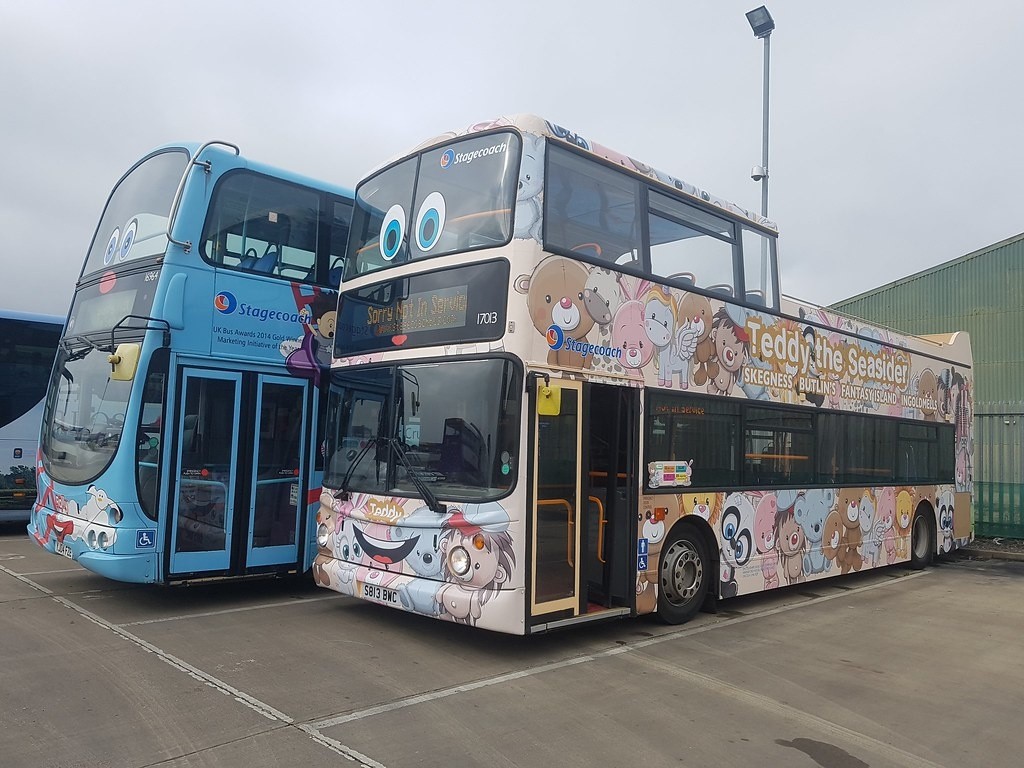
[26,140,355,589]
[314,118,982,642]
[0,311,204,523]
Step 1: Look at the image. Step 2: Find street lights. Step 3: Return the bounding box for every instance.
[747,6,777,303]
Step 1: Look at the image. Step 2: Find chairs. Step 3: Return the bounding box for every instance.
[618,443,918,491]
[236,246,258,270]
[251,241,279,274]
[326,257,345,289]
[570,242,765,308]
[182,413,199,450]
[303,264,315,282]
